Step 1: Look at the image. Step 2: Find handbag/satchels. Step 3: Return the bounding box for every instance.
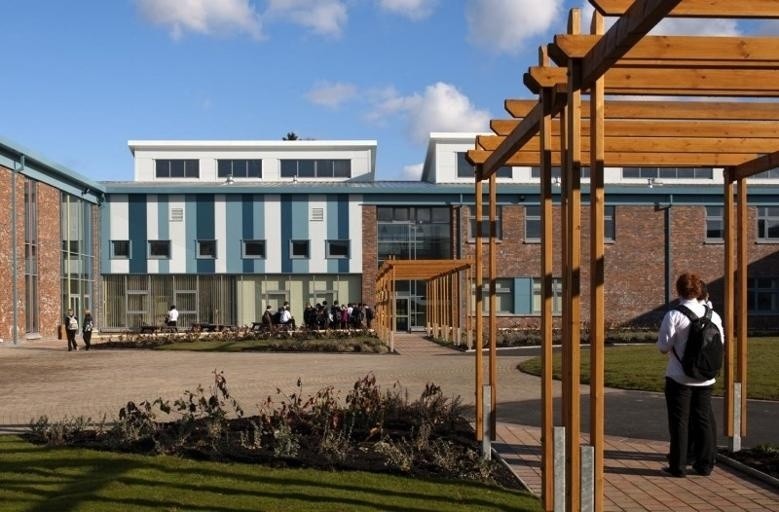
[84,321,93,332]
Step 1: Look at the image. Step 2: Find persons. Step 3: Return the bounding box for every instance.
[262,300,296,331]
[65,308,79,351]
[304,300,373,328]
[83,310,94,350]
[167,304,179,332]
[656,268,724,477]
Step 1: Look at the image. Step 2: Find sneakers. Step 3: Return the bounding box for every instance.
[661,467,684,476]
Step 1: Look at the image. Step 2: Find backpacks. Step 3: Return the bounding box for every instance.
[67,315,78,330]
[668,305,721,380]
